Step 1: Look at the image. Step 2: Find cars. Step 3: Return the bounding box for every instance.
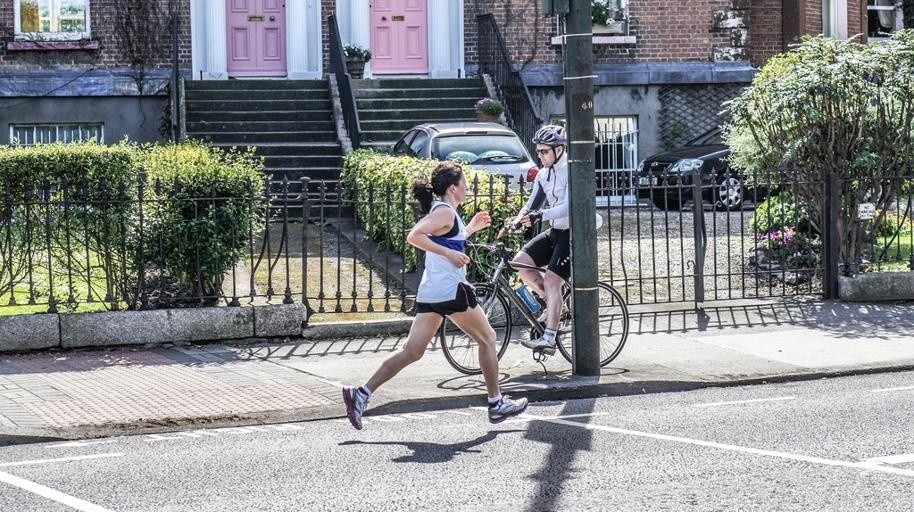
[635,124,785,209]
[393,120,540,190]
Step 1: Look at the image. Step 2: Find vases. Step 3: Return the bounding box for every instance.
[476,110,499,124]
[591,21,624,34]
[343,57,365,78]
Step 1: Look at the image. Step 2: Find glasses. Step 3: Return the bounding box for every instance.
[536,146,552,155]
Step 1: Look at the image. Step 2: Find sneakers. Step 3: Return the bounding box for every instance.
[520,336,556,356]
[342,385,368,430]
[487,395,528,424]
[536,302,569,323]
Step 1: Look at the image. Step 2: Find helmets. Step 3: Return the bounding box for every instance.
[531,125,567,145]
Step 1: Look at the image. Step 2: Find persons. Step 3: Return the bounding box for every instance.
[506,123,602,357]
[339,164,531,431]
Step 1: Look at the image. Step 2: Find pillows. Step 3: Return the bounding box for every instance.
[445,151,478,163]
[478,151,513,160]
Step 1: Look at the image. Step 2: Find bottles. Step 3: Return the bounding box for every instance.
[514,282,542,314]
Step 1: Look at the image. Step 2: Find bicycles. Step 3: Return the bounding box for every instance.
[441,242,629,369]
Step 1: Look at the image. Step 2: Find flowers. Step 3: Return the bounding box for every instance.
[474,98,503,116]
[343,41,372,62]
[591,0,613,26]
